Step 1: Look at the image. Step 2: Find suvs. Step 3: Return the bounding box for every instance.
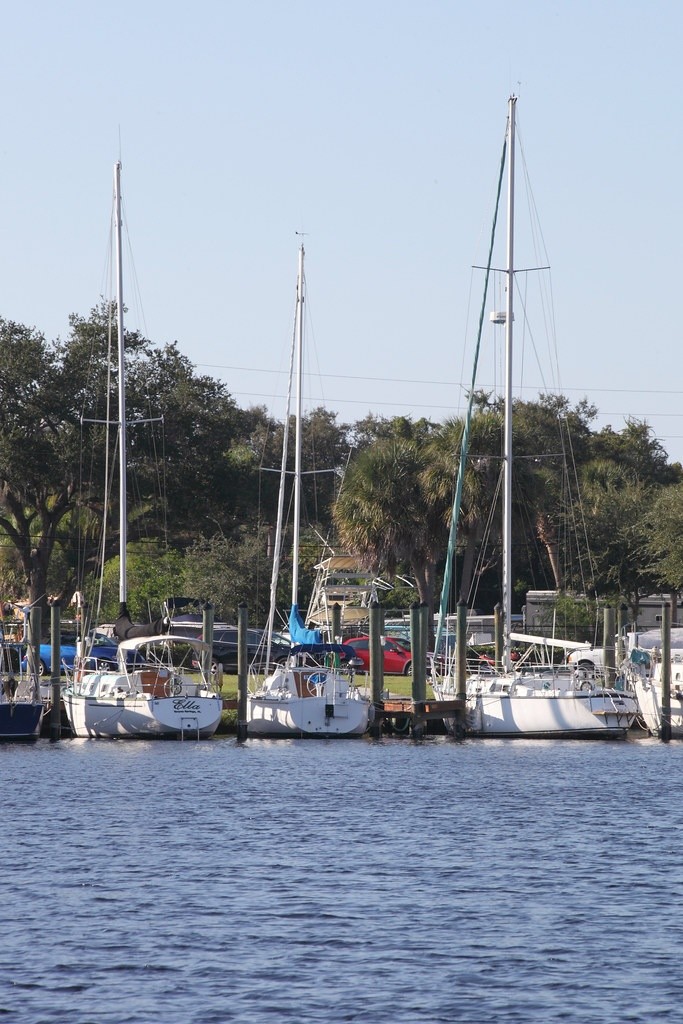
[566,628,683,691]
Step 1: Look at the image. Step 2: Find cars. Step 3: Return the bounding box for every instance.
[341,636,449,677]
[197,630,291,673]
[476,642,524,670]
[20,631,147,672]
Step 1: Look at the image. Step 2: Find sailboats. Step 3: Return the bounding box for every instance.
[245,232,375,739]
[60,163,227,740]
[429,95,681,739]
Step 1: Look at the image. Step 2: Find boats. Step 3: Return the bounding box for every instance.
[0,697,44,743]
